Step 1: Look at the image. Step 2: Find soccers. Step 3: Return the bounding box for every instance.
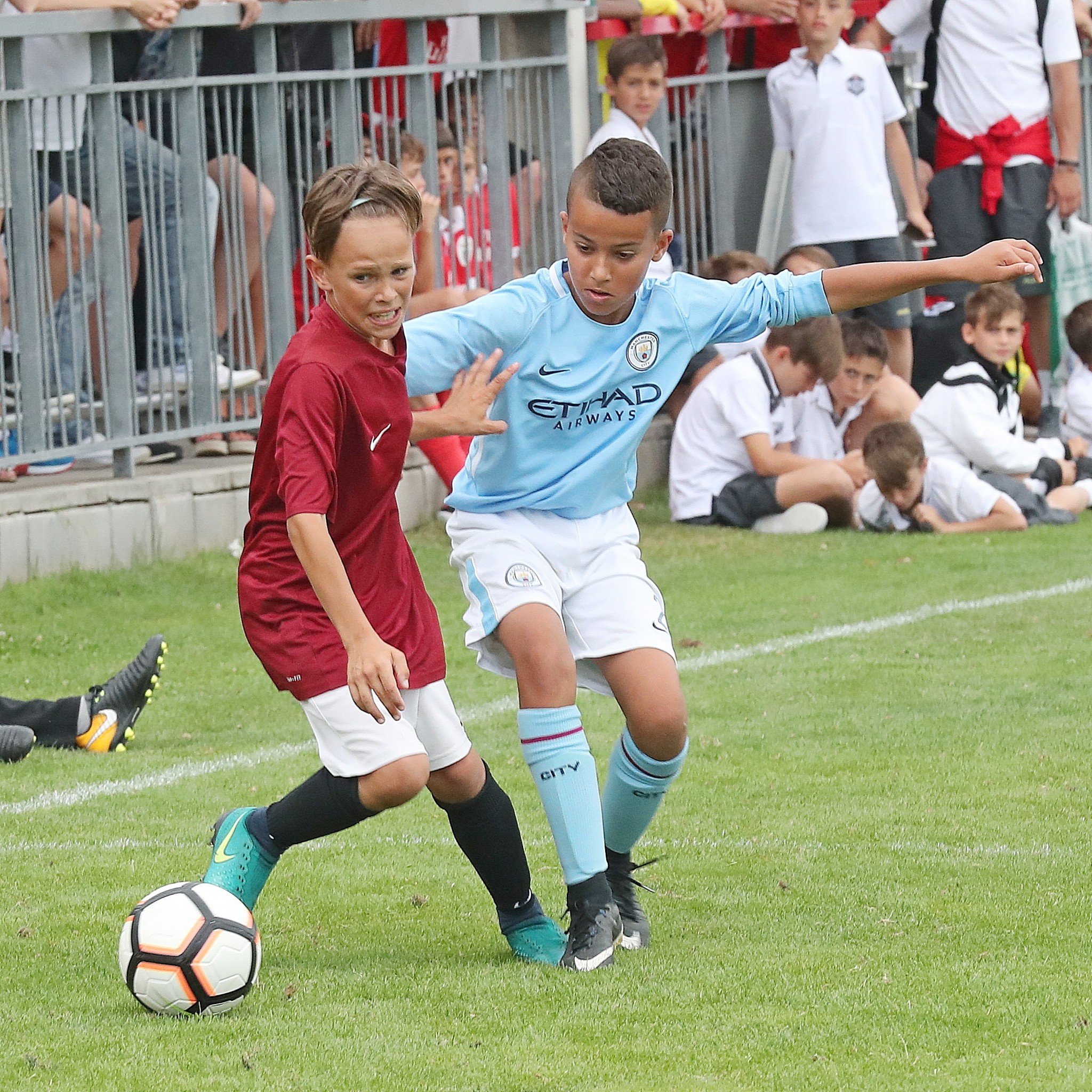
[116,880,265,1021]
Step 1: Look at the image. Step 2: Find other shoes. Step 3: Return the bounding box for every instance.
[230,430,256,454]
[196,431,229,455]
[146,350,260,393]
[752,502,829,534]
[131,441,184,463]
[1030,457,1064,492]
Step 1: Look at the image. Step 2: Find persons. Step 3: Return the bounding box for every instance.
[664,248,774,425]
[398,137,1044,971]
[585,1,1092,269]
[1056,298,1092,459]
[772,318,886,489]
[910,280,1079,496]
[1,640,169,762]
[858,0,1085,377]
[662,314,858,533]
[774,242,924,450]
[765,1,932,388]
[202,155,575,965]
[0,0,554,479]
[846,419,1092,534]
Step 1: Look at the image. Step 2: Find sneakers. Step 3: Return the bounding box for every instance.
[0,725,35,763]
[505,917,567,965]
[200,807,279,913]
[559,907,624,973]
[606,856,658,949]
[1,433,73,474]
[76,634,167,753]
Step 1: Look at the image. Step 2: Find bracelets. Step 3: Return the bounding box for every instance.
[1057,156,1080,168]
